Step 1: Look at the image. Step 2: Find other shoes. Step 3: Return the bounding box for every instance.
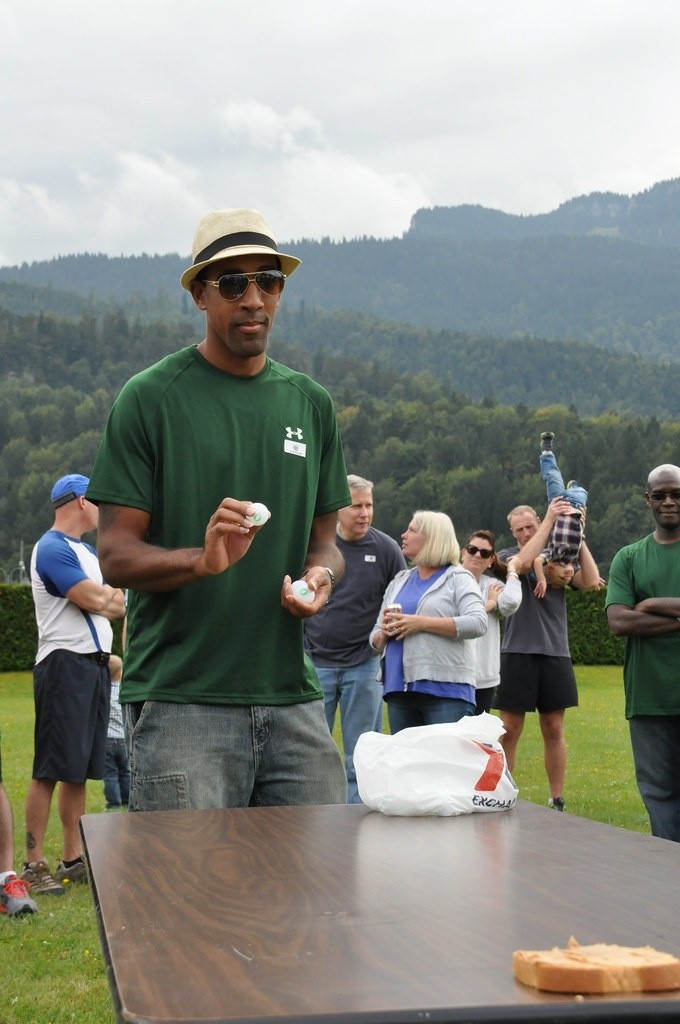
[550,799,566,813]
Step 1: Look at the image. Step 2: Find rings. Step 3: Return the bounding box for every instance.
[401,627,404,631]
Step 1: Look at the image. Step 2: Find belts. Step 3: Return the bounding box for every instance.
[82,651,110,664]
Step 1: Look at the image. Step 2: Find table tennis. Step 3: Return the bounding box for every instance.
[246,503,268,526]
[291,580,315,604]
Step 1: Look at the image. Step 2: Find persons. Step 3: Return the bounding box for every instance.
[533,432,605,598]
[460,531,523,716]
[605,464,679,843]
[103,655,130,811]
[492,506,599,811]
[22,474,126,896]
[0,749,37,918]
[84,209,353,812]
[368,511,488,735]
[303,474,408,805]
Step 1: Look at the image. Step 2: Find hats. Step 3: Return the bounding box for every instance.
[51,474,91,509]
[180,209,302,291]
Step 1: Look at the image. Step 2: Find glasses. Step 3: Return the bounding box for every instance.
[200,270,287,301]
[649,491,680,500]
[467,545,492,558]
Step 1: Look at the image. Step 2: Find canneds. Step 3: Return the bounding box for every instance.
[387,603,403,632]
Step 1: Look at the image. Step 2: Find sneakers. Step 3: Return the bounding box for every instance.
[567,480,577,489]
[55,855,88,884]
[21,861,65,895]
[540,432,555,451]
[0,875,35,915]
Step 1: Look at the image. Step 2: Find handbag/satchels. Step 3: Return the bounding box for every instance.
[353,710,518,817]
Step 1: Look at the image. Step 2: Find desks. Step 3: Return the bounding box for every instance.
[77,800,680,1024]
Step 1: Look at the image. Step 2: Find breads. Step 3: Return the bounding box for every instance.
[510,934,680,995]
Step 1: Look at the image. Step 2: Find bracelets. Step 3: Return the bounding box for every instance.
[302,568,336,608]
[506,572,518,578]
